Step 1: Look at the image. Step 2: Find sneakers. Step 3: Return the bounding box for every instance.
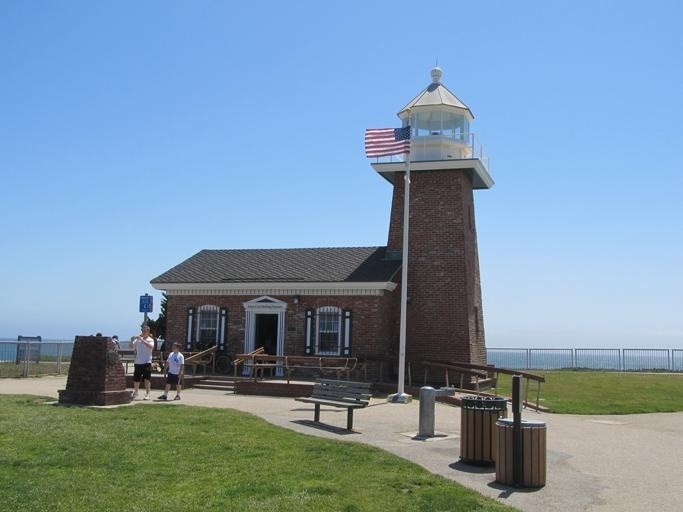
[132,390,140,401]
[172,394,181,400]
[142,392,151,400]
[156,394,168,400]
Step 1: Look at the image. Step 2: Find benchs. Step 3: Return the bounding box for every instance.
[292,373,374,433]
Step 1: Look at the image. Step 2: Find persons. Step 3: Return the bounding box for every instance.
[129,326,154,400]
[158,342,184,400]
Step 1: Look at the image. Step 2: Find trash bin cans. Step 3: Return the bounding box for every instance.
[459,395,508,466]
[495,418,546,488]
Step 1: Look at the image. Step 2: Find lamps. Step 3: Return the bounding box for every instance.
[291,295,300,304]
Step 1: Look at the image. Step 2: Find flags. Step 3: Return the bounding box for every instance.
[365,126,411,157]
